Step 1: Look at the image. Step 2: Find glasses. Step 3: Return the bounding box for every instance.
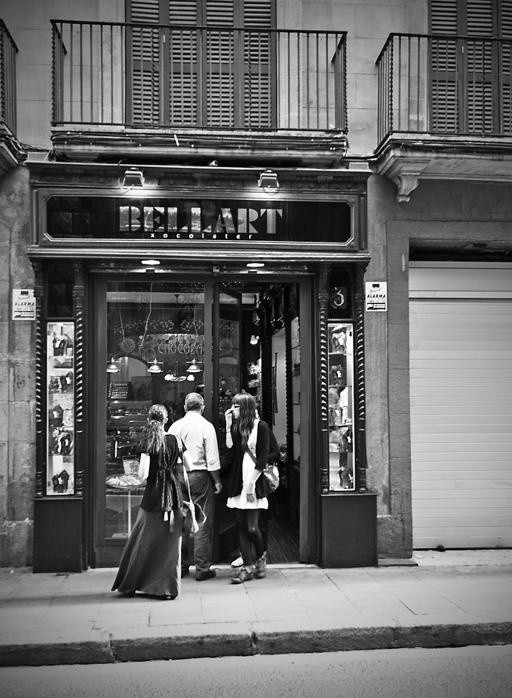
[234,405,240,409]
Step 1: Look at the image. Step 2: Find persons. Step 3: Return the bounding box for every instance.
[222,393,270,583]
[167,393,223,580]
[111,404,191,598]
[232,411,281,568]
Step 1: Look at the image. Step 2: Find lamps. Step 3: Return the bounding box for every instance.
[248,332,261,346]
[251,167,284,198]
[116,166,147,195]
[100,279,204,383]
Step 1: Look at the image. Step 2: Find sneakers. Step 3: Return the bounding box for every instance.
[231,565,254,583]
[254,554,266,579]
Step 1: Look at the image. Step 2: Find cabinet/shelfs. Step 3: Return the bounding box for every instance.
[325,315,356,493]
[246,358,262,413]
[44,319,76,496]
[288,319,300,465]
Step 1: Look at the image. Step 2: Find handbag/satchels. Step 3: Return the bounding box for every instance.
[255,464,280,499]
[181,501,207,538]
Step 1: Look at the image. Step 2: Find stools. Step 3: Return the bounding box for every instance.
[106,474,145,539]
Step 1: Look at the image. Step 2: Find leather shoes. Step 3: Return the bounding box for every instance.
[196,569,216,581]
[181,565,189,578]
